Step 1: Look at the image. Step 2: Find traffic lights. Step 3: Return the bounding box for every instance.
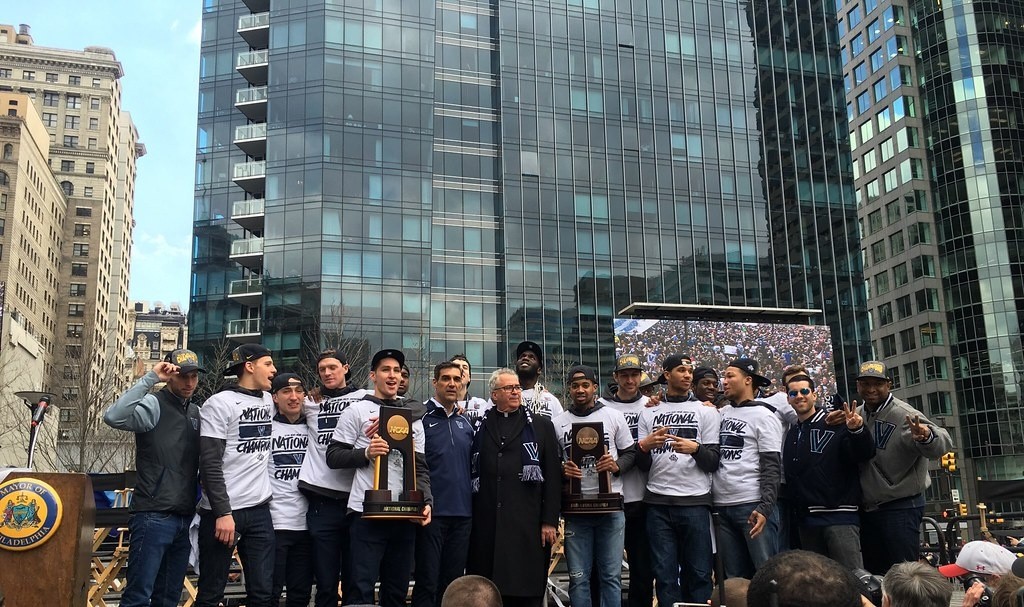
[947,452,957,474]
[941,454,951,468]
[959,503,968,516]
[943,510,952,518]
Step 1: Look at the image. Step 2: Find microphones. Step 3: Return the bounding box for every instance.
[31,395,51,427]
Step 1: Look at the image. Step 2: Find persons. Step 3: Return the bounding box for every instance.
[102,341,953,607]
[707,532,1024,607]
[615,322,837,407]
[441,575,503,607]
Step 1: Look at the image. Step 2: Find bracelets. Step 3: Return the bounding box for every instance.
[638,440,648,452]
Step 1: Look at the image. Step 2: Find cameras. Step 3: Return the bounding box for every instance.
[963,573,994,607]
[851,569,884,607]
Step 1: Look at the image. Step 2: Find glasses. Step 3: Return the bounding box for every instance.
[641,388,655,395]
[787,388,810,398]
[494,385,523,393]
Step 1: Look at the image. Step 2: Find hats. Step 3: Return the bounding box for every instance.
[568,365,599,384]
[856,361,890,380]
[272,373,303,393]
[517,341,542,362]
[938,541,1017,578]
[692,367,718,384]
[641,370,658,387]
[450,356,471,389]
[1011,556,1024,578]
[658,354,691,385]
[371,349,405,370]
[402,363,409,374]
[164,349,206,374]
[316,348,351,381]
[728,358,771,387]
[223,343,271,376]
[616,353,643,371]
